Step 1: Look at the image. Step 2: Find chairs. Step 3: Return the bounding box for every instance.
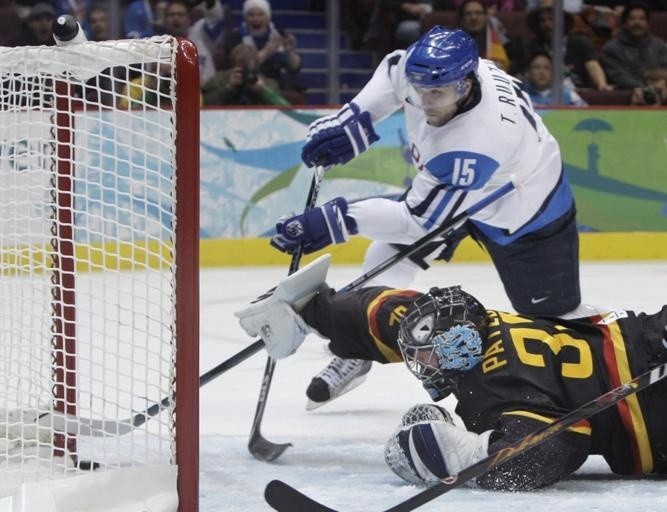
[347,1,666,106]
[1,0,317,105]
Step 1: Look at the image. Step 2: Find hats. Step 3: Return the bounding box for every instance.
[243,0,271,15]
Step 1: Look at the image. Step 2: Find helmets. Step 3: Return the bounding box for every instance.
[403,25,478,109]
[397,284,487,401]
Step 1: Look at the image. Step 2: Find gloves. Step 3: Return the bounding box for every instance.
[269,199,358,254]
[300,103,380,167]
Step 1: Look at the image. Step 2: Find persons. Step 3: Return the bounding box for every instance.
[0,1,305,110]
[340,2,666,108]
[235,253,666,493]
[268,24,581,404]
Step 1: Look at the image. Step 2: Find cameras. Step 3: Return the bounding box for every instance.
[241,66,259,84]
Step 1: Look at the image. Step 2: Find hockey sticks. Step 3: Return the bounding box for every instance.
[264,361,667,512]
[33,170,520,439]
[248,158,323,462]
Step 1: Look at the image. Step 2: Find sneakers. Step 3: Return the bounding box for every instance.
[305,353,372,402]
